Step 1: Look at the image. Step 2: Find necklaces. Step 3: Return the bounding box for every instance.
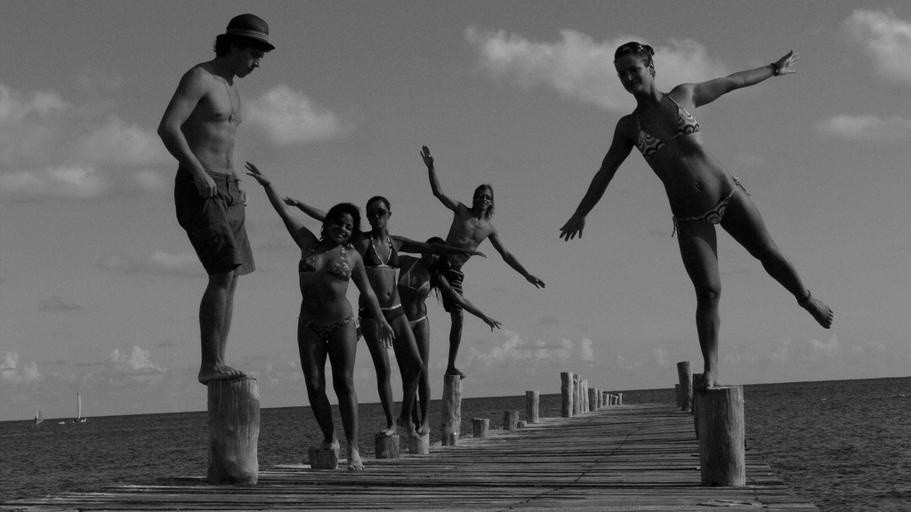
[209,58,242,124]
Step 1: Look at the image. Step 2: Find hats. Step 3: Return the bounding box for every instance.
[226,13,277,53]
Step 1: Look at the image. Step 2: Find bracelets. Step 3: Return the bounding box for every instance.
[772,61,780,77]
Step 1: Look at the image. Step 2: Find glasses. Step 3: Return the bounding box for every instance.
[367,210,391,219]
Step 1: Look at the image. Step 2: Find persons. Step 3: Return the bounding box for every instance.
[418,143,547,378]
[242,159,394,472]
[556,40,834,390]
[283,193,487,444]
[155,13,277,386]
[394,235,505,436]
